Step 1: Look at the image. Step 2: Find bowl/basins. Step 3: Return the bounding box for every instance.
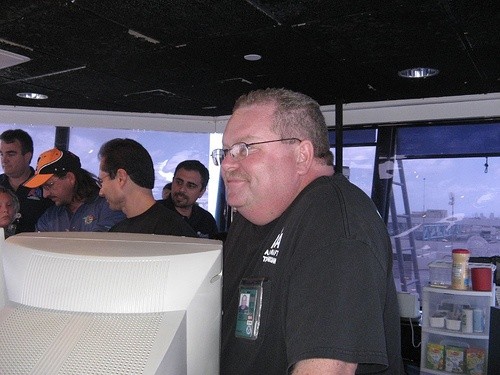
[471,267,492,292]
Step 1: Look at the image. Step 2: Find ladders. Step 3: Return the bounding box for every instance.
[389,159,422,310]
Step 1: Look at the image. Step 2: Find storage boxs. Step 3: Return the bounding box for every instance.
[428,259,497,289]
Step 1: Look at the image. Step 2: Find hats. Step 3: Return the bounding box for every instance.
[22,147,81,188]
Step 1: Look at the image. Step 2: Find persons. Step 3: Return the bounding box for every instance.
[23,146,128,232]
[95,138,198,238]
[0,129,56,234]
[0,186,21,240]
[210,87,403,375]
[154,160,220,241]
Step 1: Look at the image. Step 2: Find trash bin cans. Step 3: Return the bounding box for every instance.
[400,319,419,359]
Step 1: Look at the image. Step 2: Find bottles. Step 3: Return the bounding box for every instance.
[410,289,420,313]
[473,307,484,333]
[462,305,473,333]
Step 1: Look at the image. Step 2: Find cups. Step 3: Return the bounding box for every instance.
[451,248,471,291]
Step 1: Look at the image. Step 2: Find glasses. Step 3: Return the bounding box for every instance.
[210,138,302,166]
[95,175,110,188]
[43,177,60,191]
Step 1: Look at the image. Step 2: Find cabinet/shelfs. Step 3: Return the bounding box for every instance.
[419,287,492,375]
